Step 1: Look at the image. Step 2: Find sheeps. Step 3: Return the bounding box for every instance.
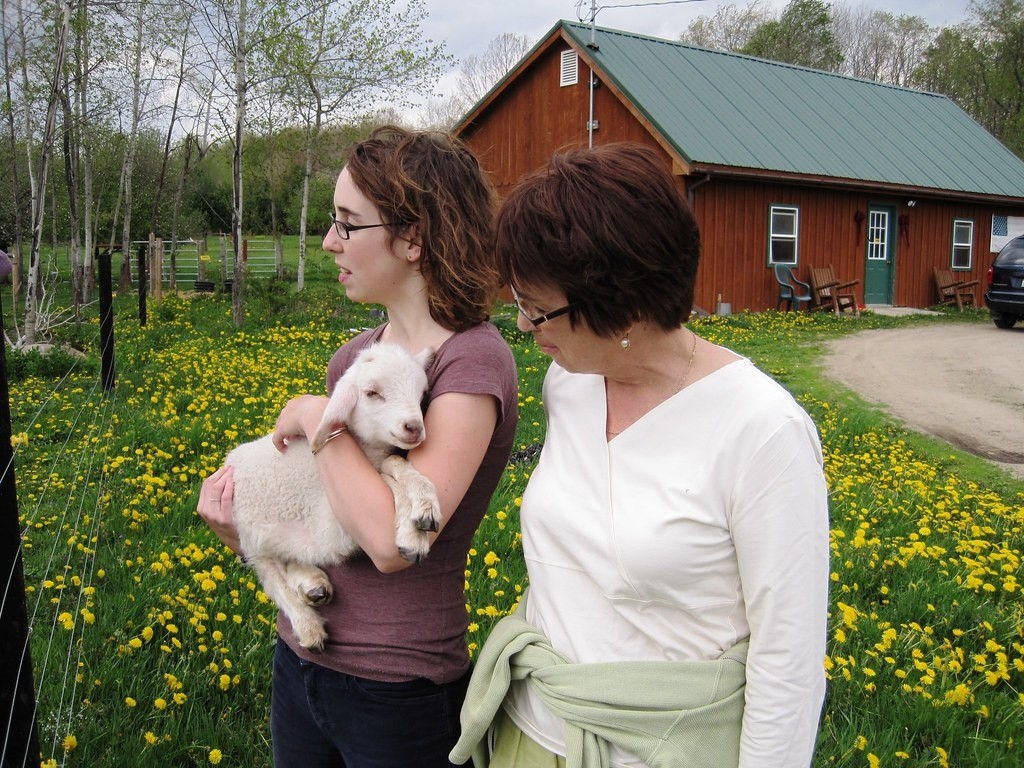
[221,343,443,655]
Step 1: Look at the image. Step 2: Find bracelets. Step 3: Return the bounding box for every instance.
[311,426,347,455]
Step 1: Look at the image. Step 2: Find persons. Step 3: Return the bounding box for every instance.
[196,126,518,768]
[448,144,830,768]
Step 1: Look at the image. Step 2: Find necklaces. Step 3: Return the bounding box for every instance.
[606,329,697,435]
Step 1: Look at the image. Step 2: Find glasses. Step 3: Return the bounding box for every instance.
[329,212,408,240]
[511,281,577,329]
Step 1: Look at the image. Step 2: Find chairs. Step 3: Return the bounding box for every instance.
[775,263,811,314]
[933,266,984,313]
[808,263,860,318]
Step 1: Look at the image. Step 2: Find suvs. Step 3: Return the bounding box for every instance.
[984,234,1024,330]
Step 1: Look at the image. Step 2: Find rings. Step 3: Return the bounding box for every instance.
[211,499,220,501]
